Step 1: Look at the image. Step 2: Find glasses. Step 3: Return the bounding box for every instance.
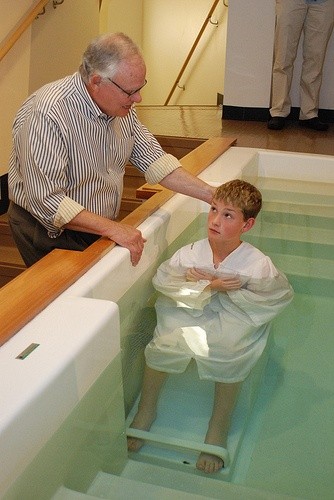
[106,76,148,97]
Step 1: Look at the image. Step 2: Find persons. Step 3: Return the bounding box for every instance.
[8,32,218,268]
[268,0,334,131]
[126,180,295,474]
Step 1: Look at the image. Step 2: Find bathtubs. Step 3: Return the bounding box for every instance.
[0,147,334,499]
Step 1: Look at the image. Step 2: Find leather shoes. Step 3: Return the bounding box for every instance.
[267,116,289,131]
[299,116,330,132]
